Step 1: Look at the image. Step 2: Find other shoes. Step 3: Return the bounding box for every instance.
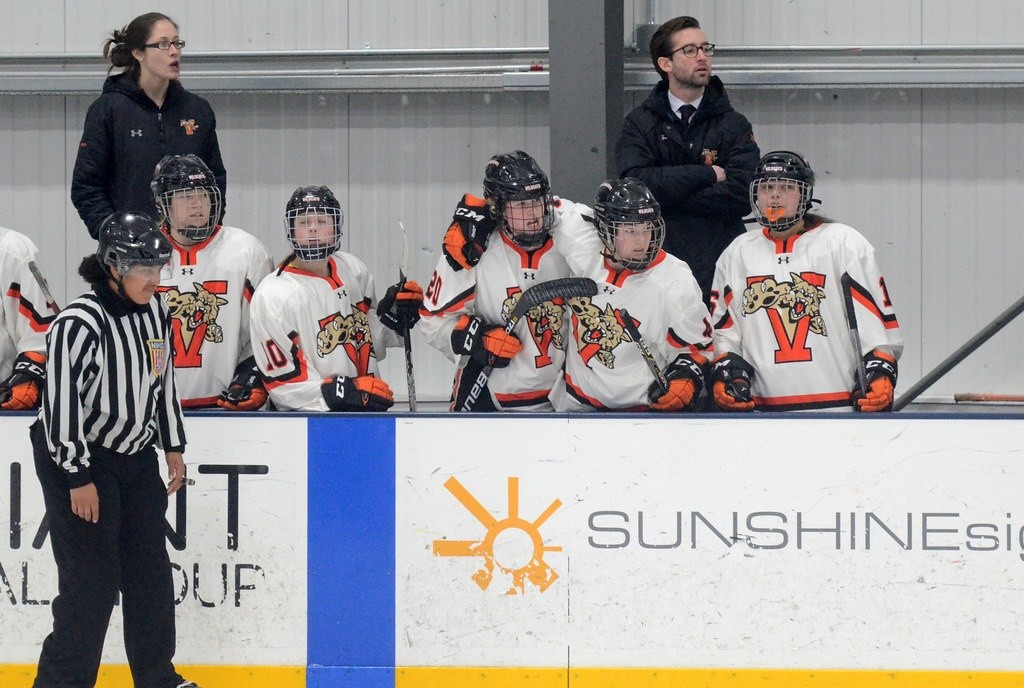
[173,675,201,688]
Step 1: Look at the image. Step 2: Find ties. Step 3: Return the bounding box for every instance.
[679,103,697,129]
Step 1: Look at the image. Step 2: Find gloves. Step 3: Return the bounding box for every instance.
[0,351,48,410]
[852,347,898,412]
[320,375,394,413]
[649,353,708,412]
[376,280,424,336]
[442,193,497,272]
[707,353,756,412]
[216,356,268,411]
[450,313,522,367]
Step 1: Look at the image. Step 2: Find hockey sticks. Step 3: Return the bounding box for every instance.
[397,219,417,411]
[459,277,599,412]
[619,308,670,395]
[28,260,61,316]
[841,274,869,398]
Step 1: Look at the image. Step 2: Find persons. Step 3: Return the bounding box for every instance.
[614,16,760,311]
[152,156,275,410]
[73,13,228,239]
[249,185,423,412]
[30,211,196,688]
[447,176,714,411]
[710,150,904,412]
[0,228,55,410]
[417,151,596,410]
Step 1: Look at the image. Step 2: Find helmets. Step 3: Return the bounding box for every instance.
[97,210,174,280]
[582,178,666,269]
[482,149,554,247]
[283,184,343,262]
[749,150,814,233]
[152,153,222,243]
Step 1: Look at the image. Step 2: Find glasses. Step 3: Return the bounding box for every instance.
[667,43,715,58]
[142,40,185,50]
